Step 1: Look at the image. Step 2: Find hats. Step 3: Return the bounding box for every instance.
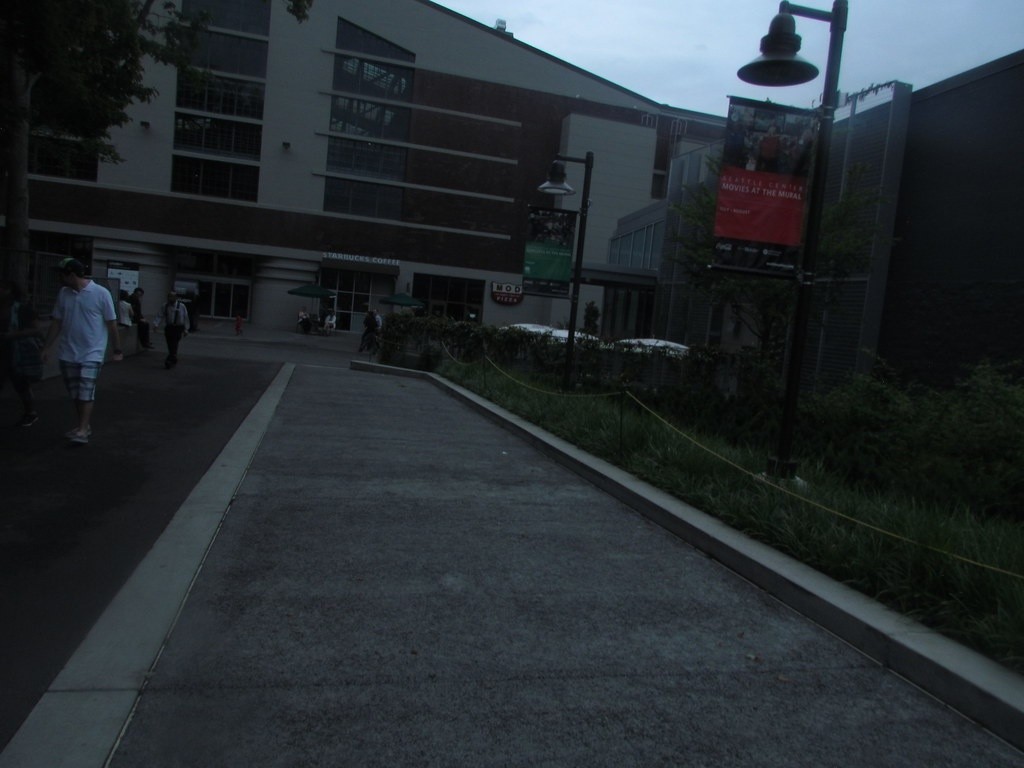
[49,258,84,273]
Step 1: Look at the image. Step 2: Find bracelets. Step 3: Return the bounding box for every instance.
[113,350,122,354]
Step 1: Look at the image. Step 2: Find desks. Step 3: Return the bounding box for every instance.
[310,320,325,337]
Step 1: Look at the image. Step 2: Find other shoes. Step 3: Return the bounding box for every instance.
[69,431,88,443]
[66,425,91,438]
[16,412,39,427]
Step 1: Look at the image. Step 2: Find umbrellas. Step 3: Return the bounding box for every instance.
[289,282,337,313]
[378,291,425,313]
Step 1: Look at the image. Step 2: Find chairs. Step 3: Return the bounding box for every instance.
[296,312,304,334]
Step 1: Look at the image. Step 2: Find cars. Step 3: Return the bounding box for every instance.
[175,281,198,330]
[616,337,688,359]
[501,322,600,347]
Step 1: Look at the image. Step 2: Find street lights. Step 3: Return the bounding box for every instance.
[537,150,593,382]
[739,0,846,473]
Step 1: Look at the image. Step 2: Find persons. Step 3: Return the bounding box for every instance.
[297,306,312,335]
[114,288,155,349]
[0,276,41,426]
[188,295,200,333]
[44,258,124,443]
[324,311,336,337]
[152,292,190,367]
[359,309,382,352]
